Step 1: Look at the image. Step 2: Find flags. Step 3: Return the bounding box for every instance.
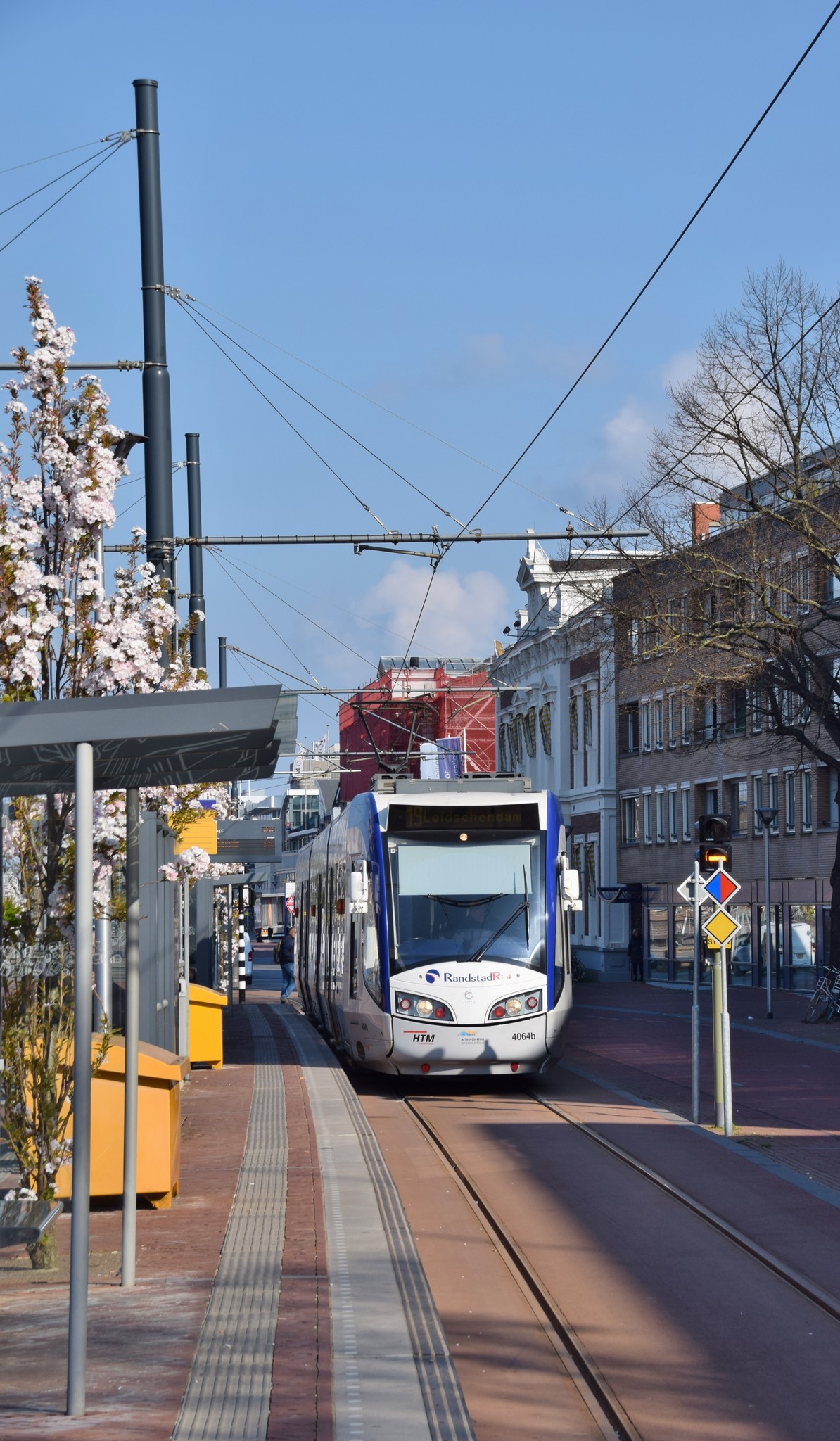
[420,737,464,780]
[315,727,328,761]
[292,748,304,778]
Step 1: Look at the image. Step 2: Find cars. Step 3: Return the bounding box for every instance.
[244,932,254,985]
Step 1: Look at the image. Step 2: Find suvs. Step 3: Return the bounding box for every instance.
[732,922,816,976]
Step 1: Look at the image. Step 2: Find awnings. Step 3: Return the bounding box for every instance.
[498,691,593,771]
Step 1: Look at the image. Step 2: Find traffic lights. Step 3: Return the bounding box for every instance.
[699,815,732,841]
[699,845,733,872]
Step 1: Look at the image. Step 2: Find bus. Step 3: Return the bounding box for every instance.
[293,772,583,1076]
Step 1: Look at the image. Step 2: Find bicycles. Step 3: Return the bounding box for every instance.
[804,966,840,1023]
[571,949,585,985]
[825,973,840,1024]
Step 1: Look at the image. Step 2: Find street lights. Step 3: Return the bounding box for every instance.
[752,809,783,1019]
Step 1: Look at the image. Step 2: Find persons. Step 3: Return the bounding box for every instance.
[189,966,196,983]
[627,928,644,982]
[452,903,504,954]
[279,927,296,1005]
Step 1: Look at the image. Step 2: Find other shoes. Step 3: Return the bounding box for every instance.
[639,975,643,981]
[281,997,294,1006]
[633,975,638,981]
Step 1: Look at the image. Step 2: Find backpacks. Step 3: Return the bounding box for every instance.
[273,936,293,964]
